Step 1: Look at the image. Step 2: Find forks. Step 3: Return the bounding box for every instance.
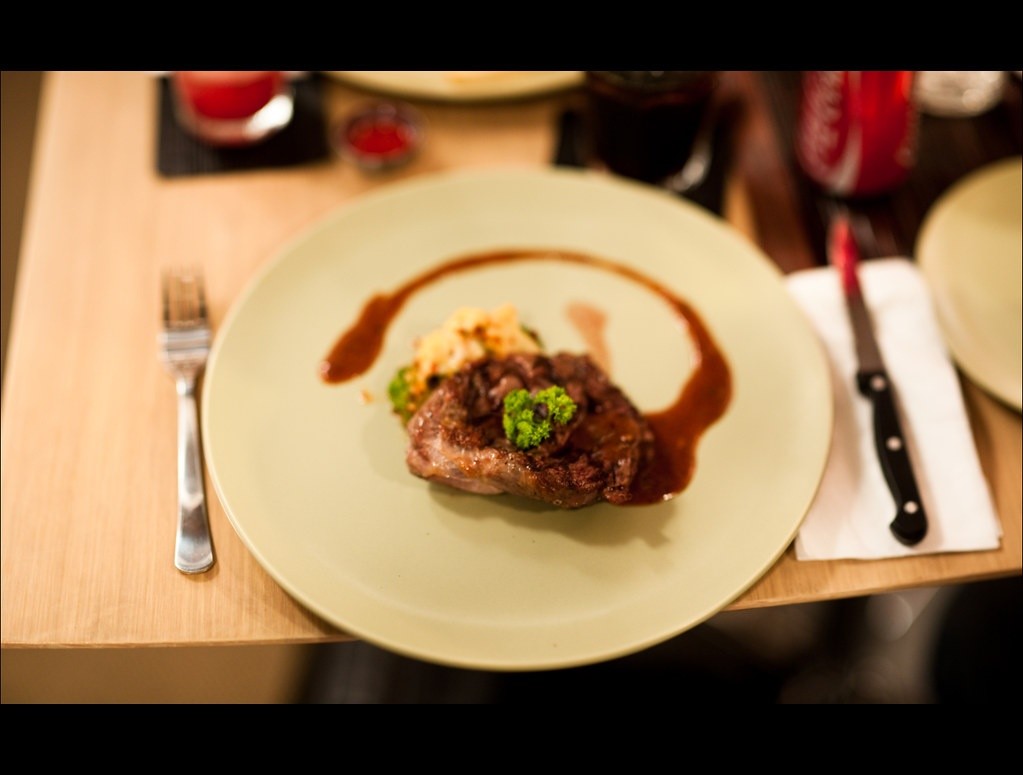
[158,267,223,573]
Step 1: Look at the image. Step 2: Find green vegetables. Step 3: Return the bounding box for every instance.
[388,367,431,424]
[500,386,578,449]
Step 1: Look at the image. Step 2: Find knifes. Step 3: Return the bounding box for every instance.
[845,264,928,548]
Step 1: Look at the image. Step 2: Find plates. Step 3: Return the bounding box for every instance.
[913,159,1023,414]
[202,164,838,672]
[321,71,584,103]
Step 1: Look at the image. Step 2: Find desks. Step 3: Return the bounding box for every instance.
[1,72,1022,704]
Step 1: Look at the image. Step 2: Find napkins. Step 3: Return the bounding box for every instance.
[783,258,1005,562]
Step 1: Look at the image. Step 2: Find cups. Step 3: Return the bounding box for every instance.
[582,71,723,194]
[913,70,1009,119]
[169,71,296,147]
